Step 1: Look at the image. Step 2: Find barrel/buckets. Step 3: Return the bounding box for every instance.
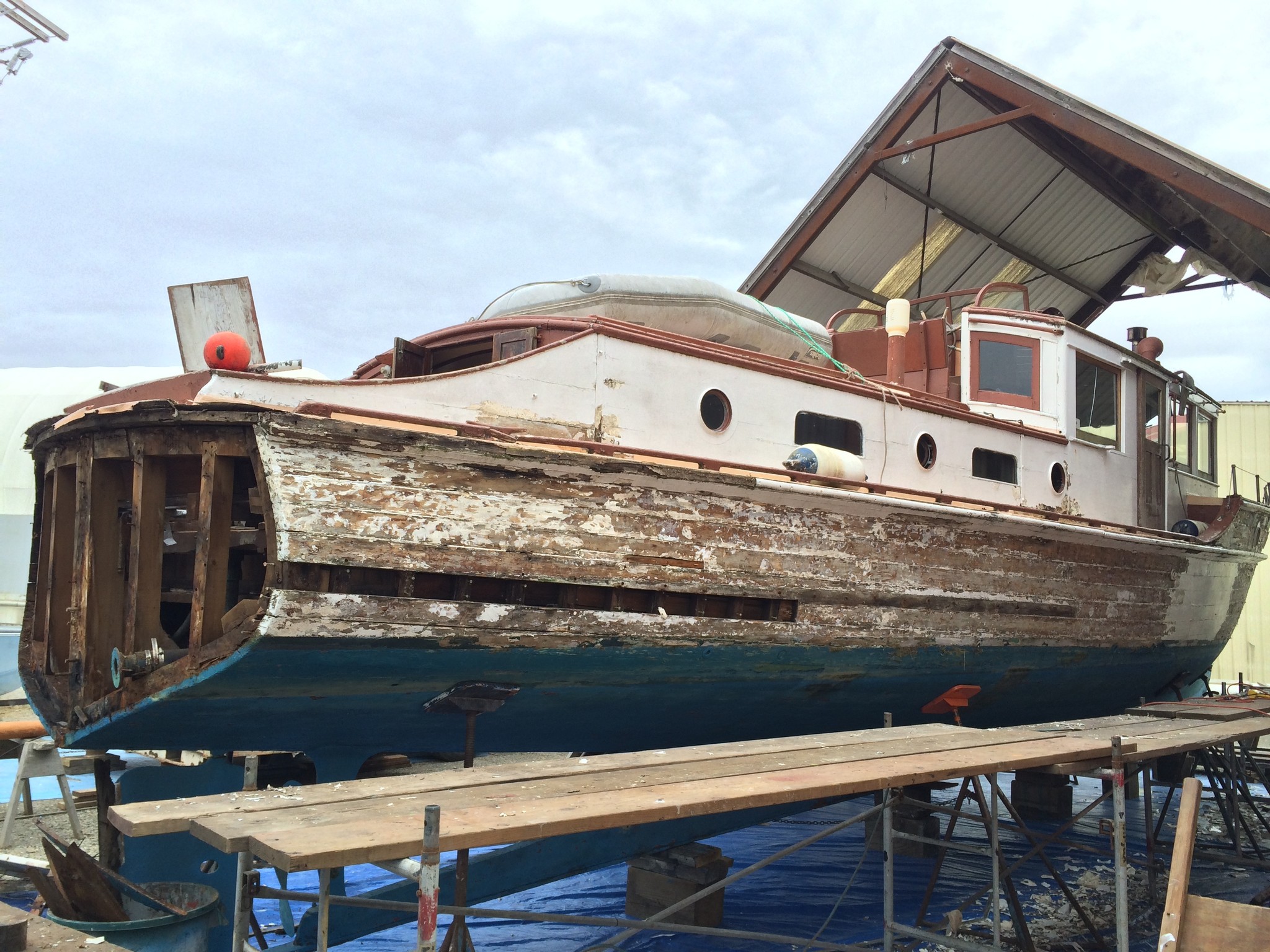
[46,881,219,951]
[1171,520,1211,541]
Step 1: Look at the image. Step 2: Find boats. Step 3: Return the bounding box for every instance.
[23,278,1270,755]
[475,276,832,370]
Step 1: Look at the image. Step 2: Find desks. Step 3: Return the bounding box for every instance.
[106,713,1142,952]
[1028,688,1270,952]
